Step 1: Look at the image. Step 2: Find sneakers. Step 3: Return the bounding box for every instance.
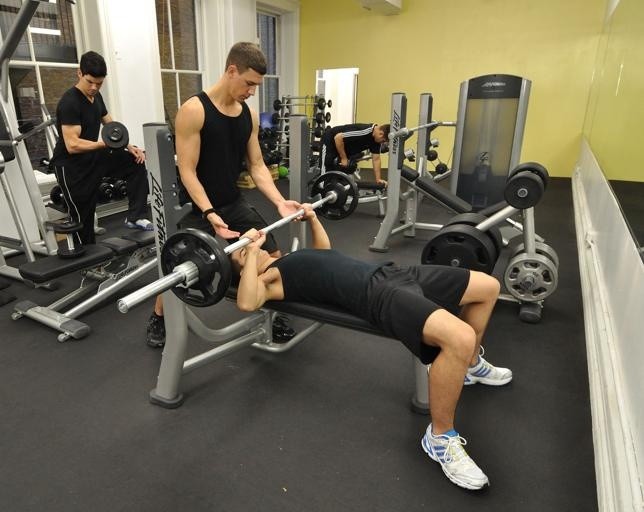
[127,219,154,231]
[273,316,296,342]
[146,312,165,348]
[463,354,512,386]
[421,423,490,490]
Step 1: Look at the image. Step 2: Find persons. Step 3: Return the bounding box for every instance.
[145,41,304,348]
[51,52,153,251]
[320,123,391,188]
[226,203,513,491]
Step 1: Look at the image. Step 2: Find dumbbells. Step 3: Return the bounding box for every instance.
[53,220,86,259]
[257,125,278,166]
[50,177,129,206]
[101,121,128,155]
[332,157,357,174]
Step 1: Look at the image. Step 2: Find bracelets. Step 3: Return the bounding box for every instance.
[201,208,215,218]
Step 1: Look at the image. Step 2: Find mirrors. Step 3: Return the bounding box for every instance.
[583,1,644,266]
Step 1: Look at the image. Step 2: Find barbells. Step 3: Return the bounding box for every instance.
[271,98,332,168]
[116,172,359,313]
